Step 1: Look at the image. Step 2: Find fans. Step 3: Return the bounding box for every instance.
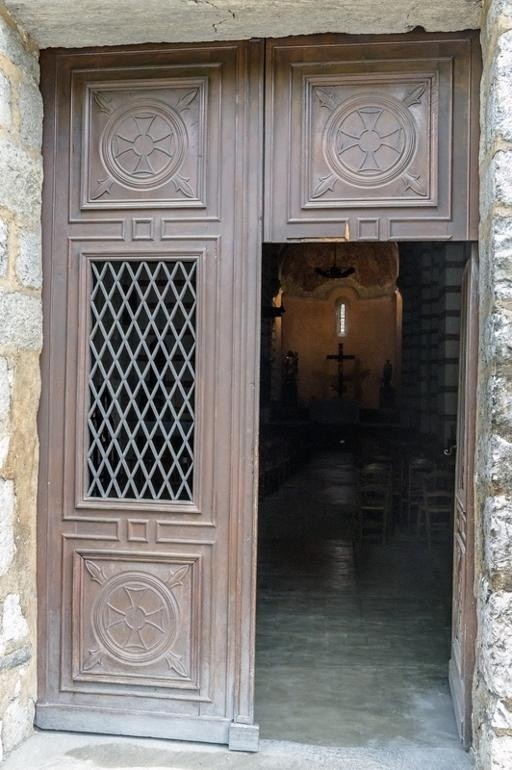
[315,244,355,279]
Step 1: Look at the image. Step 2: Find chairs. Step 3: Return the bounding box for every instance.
[346,428,456,553]
